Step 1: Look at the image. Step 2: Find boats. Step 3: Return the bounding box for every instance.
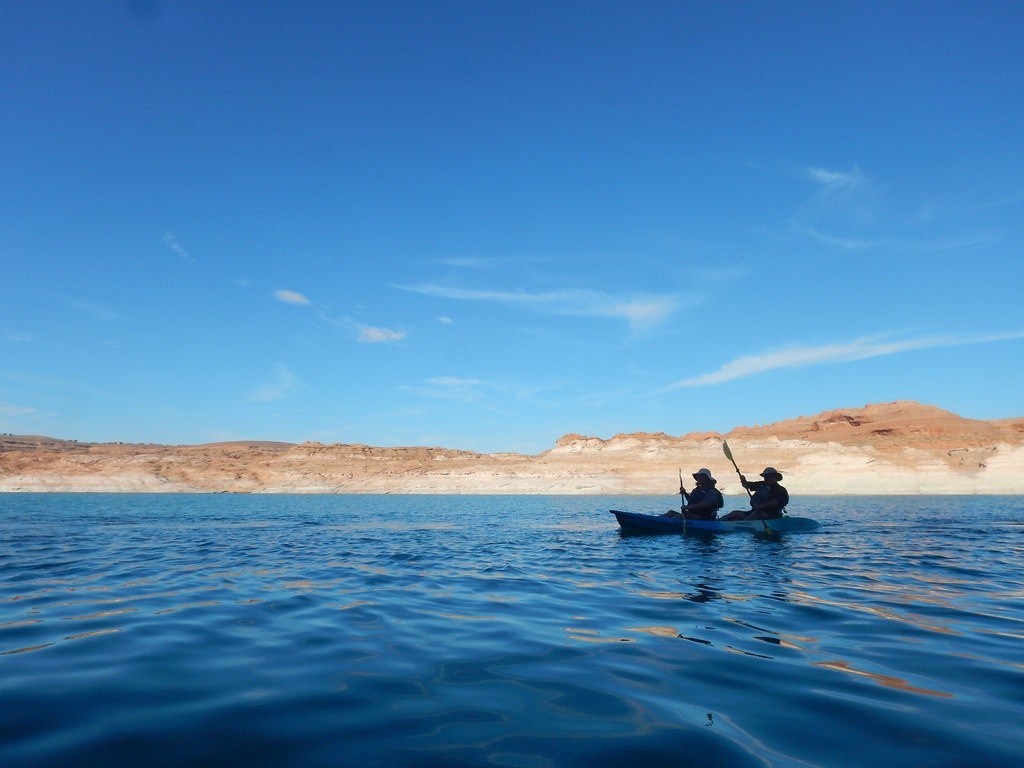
[607,508,820,535]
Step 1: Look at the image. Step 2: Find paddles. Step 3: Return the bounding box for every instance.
[678,467,689,540]
[723,441,781,539]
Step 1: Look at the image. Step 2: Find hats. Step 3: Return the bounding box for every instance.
[692,468,716,484]
[759,467,783,481]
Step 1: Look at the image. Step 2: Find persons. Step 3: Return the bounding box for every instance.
[666,468,724,519]
[719,467,789,521]
